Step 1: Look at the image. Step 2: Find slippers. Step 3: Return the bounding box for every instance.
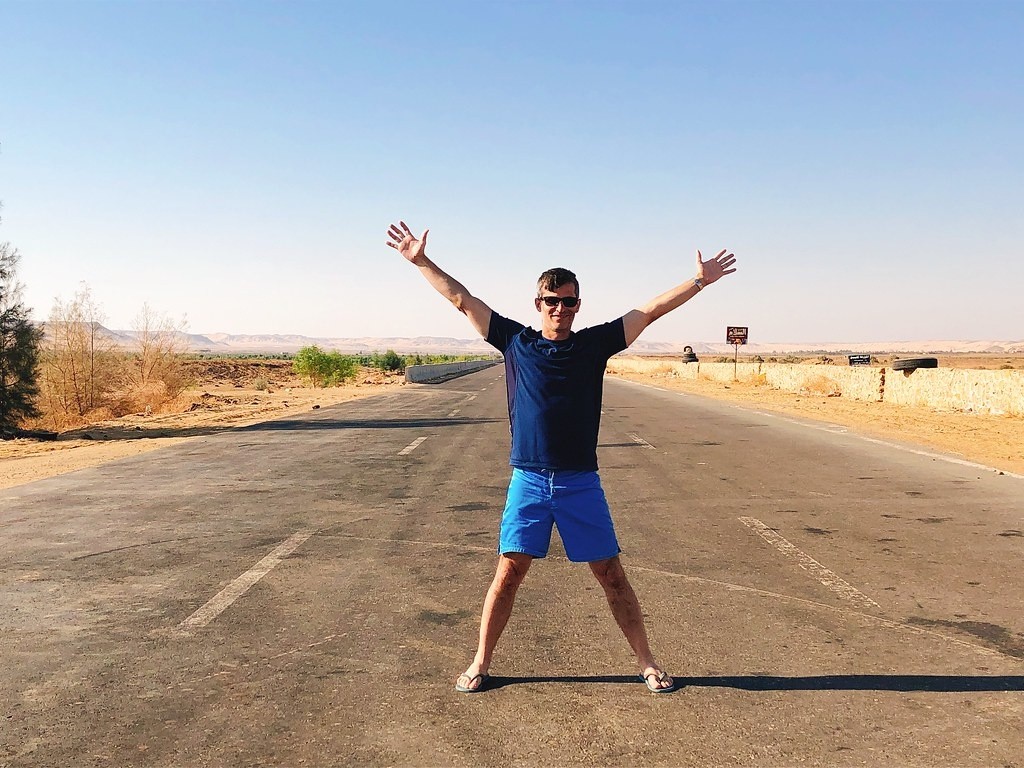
[456,673,490,693]
[639,671,674,693]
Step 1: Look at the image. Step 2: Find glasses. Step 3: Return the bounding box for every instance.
[539,296,579,307]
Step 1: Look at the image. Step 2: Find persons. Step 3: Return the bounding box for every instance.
[388,221,737,692]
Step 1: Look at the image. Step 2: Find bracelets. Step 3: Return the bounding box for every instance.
[693,278,704,290]
